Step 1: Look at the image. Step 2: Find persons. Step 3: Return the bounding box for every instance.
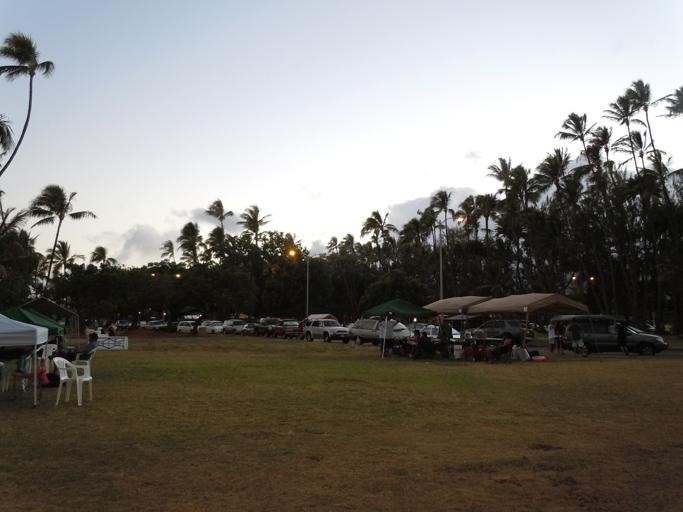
[616,322,628,358]
[567,317,585,357]
[546,320,558,353]
[493,331,513,361]
[554,321,566,355]
[47,331,97,375]
[405,330,419,346]
[379,313,399,358]
[413,331,429,352]
[464,333,480,361]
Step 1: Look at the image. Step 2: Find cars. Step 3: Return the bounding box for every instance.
[116,320,129,328]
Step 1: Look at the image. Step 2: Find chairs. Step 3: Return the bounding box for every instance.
[381,338,519,362]
[0,344,97,409]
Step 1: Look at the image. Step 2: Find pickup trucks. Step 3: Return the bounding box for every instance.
[144,320,167,330]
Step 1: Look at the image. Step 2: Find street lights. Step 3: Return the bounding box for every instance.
[289,250,310,316]
[439,215,464,300]
